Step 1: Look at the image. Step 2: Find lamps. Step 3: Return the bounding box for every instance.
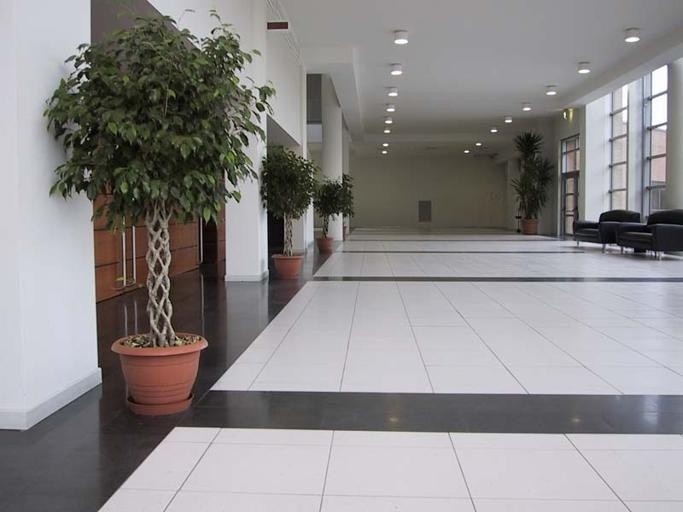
[376,28,646,155]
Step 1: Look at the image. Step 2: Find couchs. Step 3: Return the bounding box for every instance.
[612,207,682,261]
[569,206,641,255]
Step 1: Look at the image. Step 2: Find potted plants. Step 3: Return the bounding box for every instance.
[255,139,329,284]
[506,130,558,236]
[341,172,355,241]
[41,6,277,419]
[310,171,354,255]
